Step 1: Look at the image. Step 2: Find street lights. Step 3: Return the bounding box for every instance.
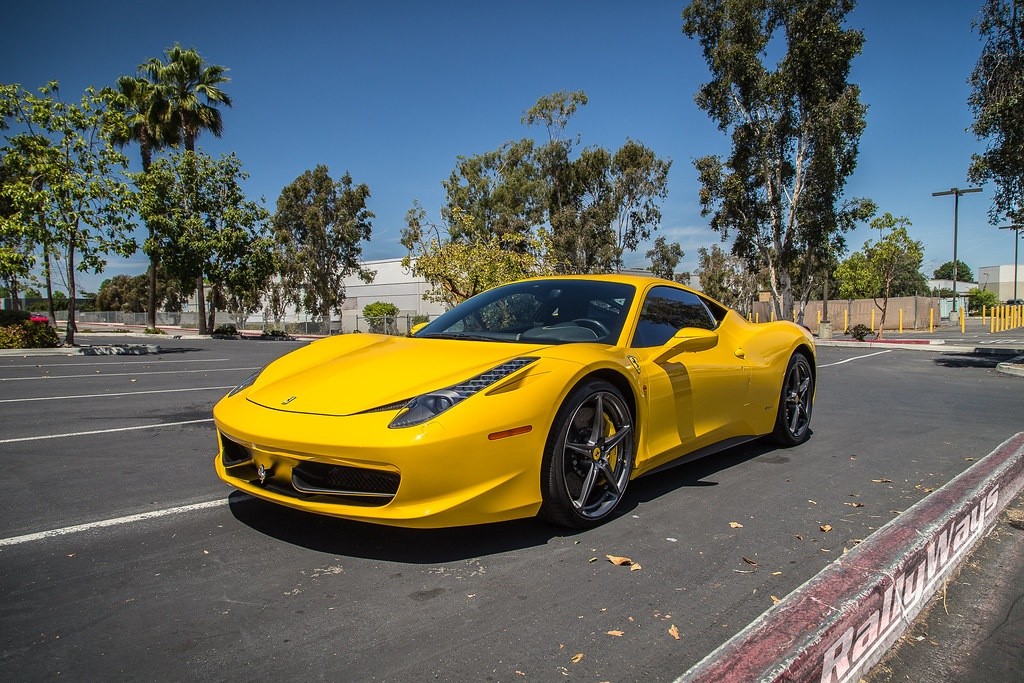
[998,225,1024,305]
[932,187,984,322]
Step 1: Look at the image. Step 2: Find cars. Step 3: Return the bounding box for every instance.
[28,312,50,325]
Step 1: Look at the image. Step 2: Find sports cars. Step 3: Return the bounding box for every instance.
[210,266,817,531]
